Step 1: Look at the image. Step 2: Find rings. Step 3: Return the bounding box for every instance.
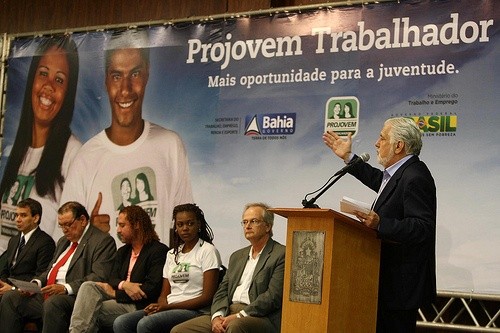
[362,218,365,222]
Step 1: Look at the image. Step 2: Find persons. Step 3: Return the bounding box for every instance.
[323,117,438,333]
[0,198,286,333]
[330,102,354,119]
[0,26,195,248]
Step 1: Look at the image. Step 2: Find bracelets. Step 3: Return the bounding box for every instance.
[11,285,15,290]
[64,287,67,292]
[236,313,241,318]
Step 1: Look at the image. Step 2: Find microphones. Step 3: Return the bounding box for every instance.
[334,152,370,176]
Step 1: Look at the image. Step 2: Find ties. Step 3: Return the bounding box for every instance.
[44,242,78,300]
[16,236,27,264]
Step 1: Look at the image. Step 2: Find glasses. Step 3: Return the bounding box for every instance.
[58,215,78,229]
[241,219,268,227]
